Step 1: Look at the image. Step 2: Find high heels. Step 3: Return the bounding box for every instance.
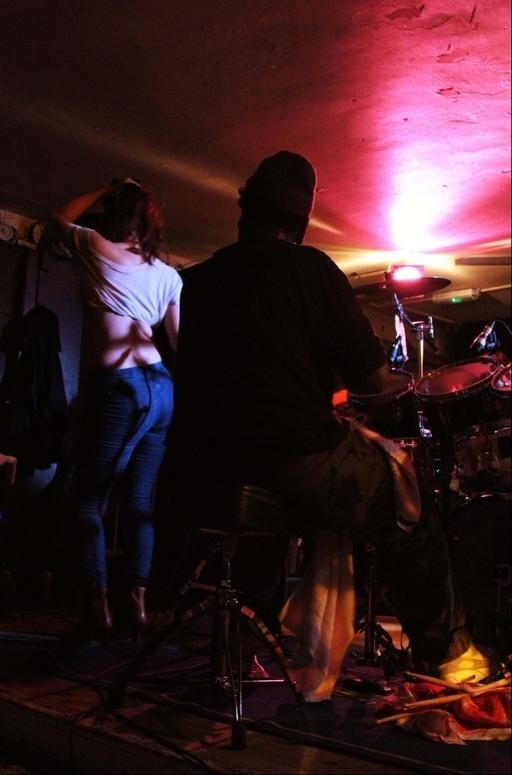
[112,585,147,646]
[60,588,113,646]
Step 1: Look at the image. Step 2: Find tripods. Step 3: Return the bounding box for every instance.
[352,557,410,677]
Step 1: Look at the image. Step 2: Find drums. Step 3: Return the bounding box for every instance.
[490,361,511,419]
[451,419,512,498]
[413,353,512,462]
[393,410,442,487]
[347,367,420,437]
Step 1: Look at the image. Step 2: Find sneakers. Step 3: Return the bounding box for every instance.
[438,641,502,685]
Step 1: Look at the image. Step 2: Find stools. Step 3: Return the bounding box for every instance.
[101,482,310,752]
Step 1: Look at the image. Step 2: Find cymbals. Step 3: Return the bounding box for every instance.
[453,258,510,266]
[354,278,450,298]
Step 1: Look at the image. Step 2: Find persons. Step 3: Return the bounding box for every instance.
[164,151,503,687]
[52,171,181,654]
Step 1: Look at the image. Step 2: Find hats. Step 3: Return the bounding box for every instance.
[241,150,318,218]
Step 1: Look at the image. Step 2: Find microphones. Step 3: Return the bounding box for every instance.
[475,319,496,356]
[389,335,401,364]
